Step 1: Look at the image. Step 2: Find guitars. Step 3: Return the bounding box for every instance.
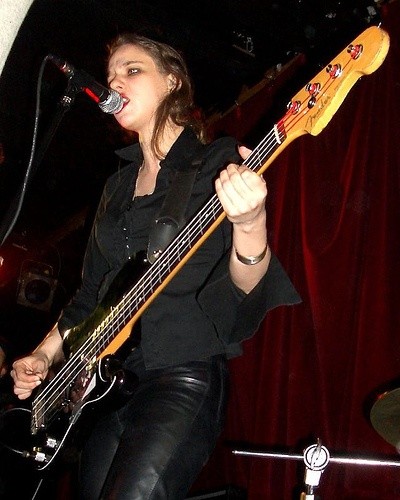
[1,24,391,483]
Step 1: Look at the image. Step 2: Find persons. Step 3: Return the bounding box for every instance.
[7,36,302,500]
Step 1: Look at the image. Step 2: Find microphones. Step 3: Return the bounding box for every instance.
[48,54,123,114]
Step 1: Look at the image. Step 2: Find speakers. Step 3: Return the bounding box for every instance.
[17,272,58,311]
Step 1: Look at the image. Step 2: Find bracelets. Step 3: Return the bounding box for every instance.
[232,241,268,266]
